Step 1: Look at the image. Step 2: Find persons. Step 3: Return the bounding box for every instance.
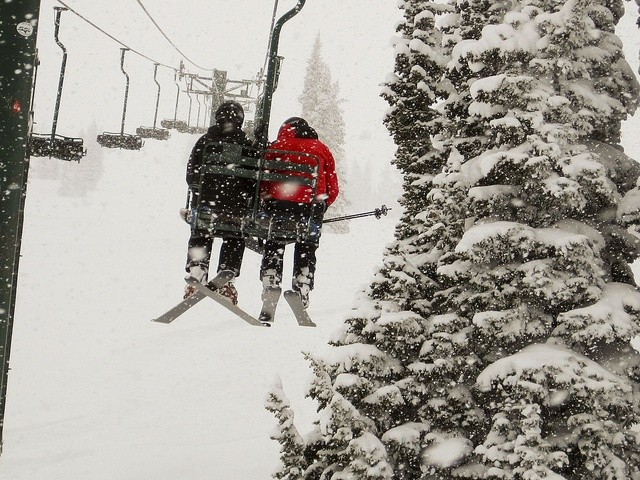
[184,101,260,306]
[260,117,339,309]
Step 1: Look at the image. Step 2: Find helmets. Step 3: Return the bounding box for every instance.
[215,100,245,128]
[279,117,309,136]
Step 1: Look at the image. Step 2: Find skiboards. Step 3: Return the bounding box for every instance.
[153,270,265,326]
[259,285,317,327]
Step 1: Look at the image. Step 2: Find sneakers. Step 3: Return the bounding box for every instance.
[259,276,280,301]
[217,281,238,306]
[183,284,198,299]
[292,280,309,310]
